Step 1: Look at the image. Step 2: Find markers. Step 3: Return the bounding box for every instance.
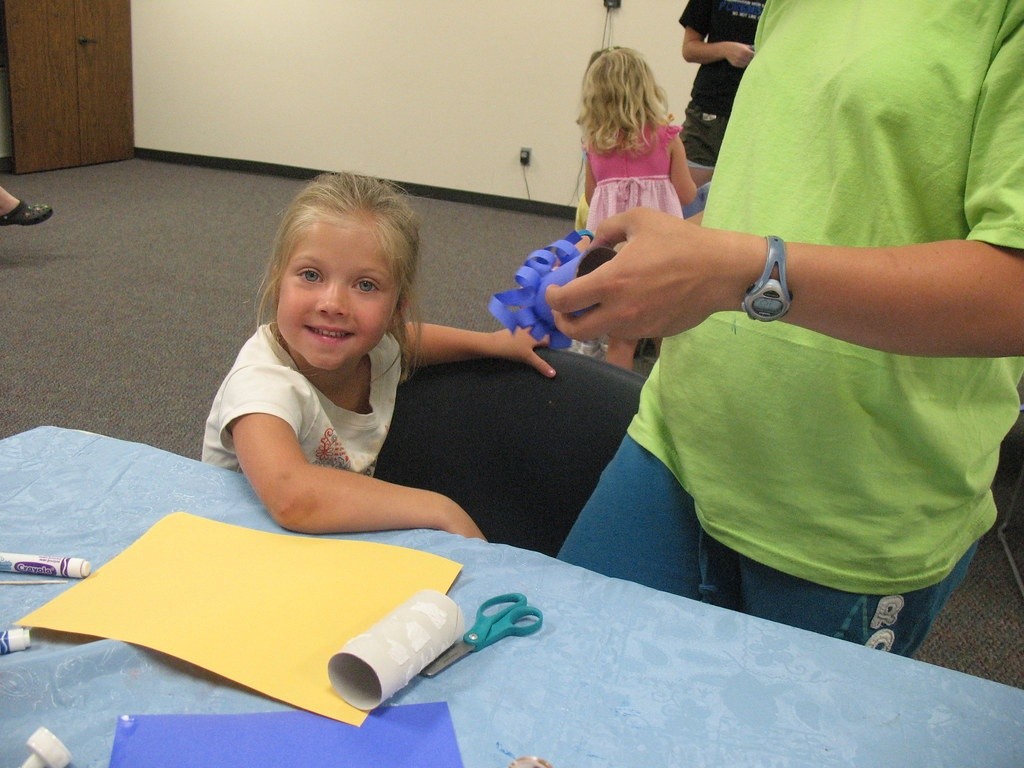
[0,627,31,656]
[0,552,91,579]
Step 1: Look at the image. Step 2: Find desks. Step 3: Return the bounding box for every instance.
[0,425,1024,768]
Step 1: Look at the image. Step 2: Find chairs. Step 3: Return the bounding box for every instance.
[372,347,650,556]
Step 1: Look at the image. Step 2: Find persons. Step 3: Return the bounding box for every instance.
[573,45,697,373]
[544,0,1024,656]
[0,186,52,227]
[201,170,556,547]
[679,0,766,191]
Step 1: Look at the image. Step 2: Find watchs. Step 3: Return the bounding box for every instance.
[742,235,794,323]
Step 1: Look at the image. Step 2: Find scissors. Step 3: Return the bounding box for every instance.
[419,592,543,679]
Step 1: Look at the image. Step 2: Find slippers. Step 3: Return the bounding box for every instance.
[0,200,53,225]
